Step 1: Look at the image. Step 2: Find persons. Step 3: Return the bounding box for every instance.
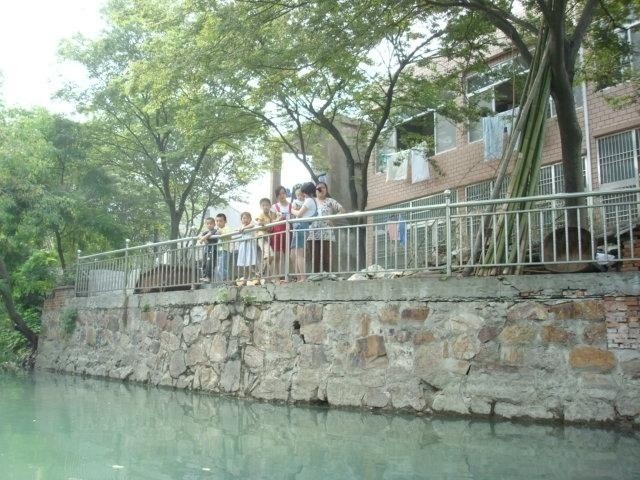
[194,181,347,290]
